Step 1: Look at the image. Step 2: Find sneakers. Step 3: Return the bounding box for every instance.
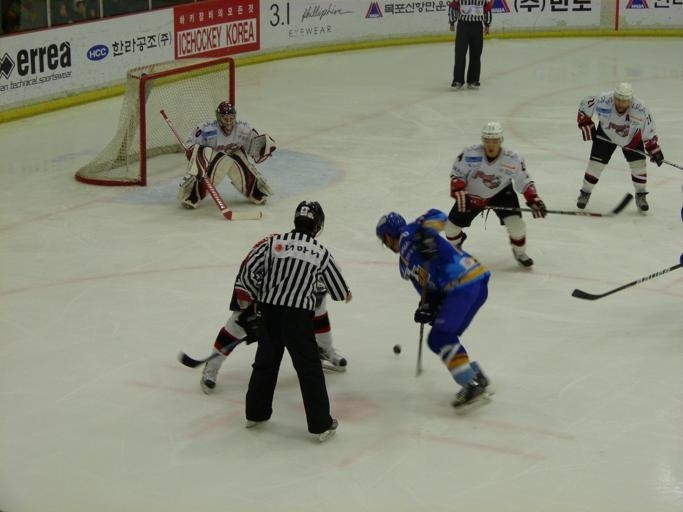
[327,419,338,430]
[452,372,489,409]
[451,80,481,87]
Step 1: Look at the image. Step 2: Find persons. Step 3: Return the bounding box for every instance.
[235,202,351,434]
[443,120,545,268]
[375,208,490,409]
[202,233,347,389]
[0,0,97,35]
[179,100,270,209]
[448,0,492,89]
[577,83,664,212]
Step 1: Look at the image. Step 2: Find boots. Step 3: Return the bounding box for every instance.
[201,362,219,389]
[513,248,534,267]
[576,189,592,209]
[635,191,650,212]
[318,346,348,367]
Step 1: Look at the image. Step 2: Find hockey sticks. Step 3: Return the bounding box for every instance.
[484,194,632,218]
[160,111,262,221]
[177,338,249,369]
[572,265,681,299]
[323,362,344,372]
[415,322,425,376]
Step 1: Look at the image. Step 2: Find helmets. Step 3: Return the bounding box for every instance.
[613,83,633,102]
[481,121,505,144]
[294,200,326,234]
[215,101,236,129]
[376,212,407,243]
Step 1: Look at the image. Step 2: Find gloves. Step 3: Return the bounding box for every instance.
[645,142,664,167]
[414,295,441,324]
[579,119,597,141]
[416,228,439,262]
[526,193,547,219]
[467,193,486,211]
